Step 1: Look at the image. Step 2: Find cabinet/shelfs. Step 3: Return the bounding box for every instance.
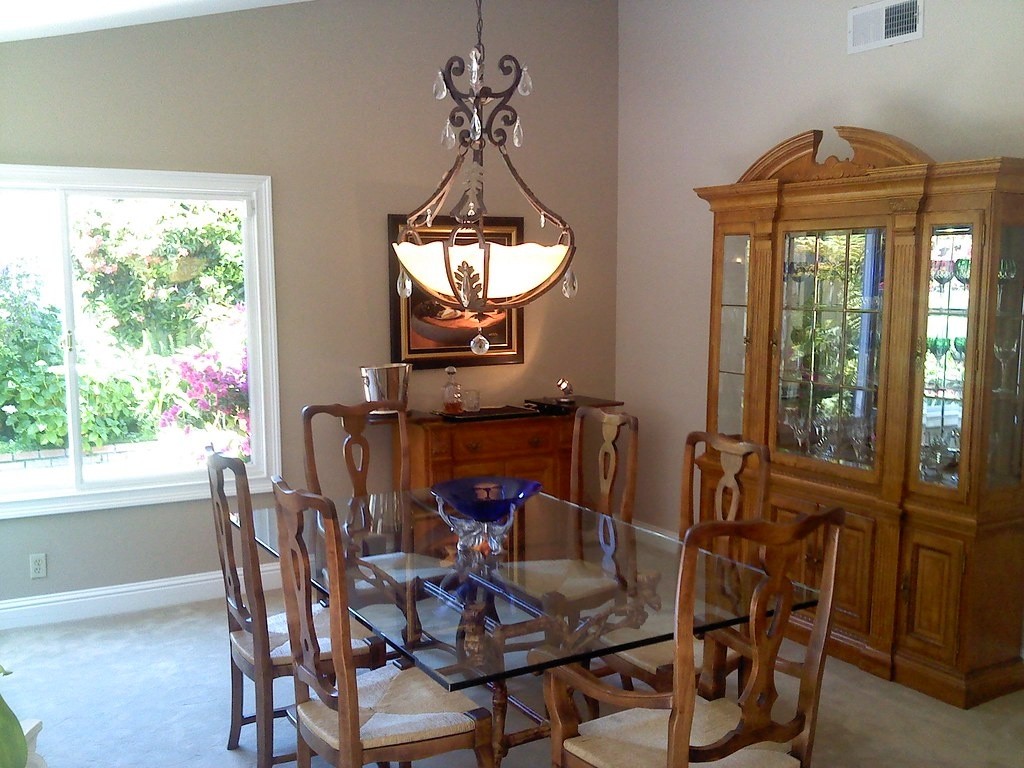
[694,127,1024,709]
[364,395,625,562]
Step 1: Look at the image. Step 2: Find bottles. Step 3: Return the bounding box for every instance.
[440,366,463,413]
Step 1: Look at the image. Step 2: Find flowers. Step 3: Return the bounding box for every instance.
[161,305,252,465]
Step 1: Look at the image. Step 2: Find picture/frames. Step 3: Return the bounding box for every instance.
[387,214,525,370]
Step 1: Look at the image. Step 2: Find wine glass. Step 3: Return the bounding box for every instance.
[790,260,861,308]
[922,429,962,487]
[784,402,875,463]
[955,258,971,292]
[997,258,1018,313]
[994,337,1018,394]
[933,260,952,292]
[954,336,968,365]
[790,325,810,372]
[925,338,950,368]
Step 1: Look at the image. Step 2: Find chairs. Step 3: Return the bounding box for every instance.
[270,473,496,768]
[527,506,845,768]
[301,403,463,687]
[491,408,639,676]
[582,430,770,721]
[205,453,392,768]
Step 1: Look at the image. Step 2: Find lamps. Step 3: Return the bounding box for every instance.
[391,0,578,354]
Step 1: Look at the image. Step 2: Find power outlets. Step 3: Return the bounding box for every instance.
[30,553,47,578]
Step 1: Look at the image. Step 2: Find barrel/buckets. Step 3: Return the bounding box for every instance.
[359,363,411,413]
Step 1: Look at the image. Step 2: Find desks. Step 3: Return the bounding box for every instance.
[226,476,820,768]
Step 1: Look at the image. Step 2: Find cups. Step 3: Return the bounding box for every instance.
[463,389,480,411]
